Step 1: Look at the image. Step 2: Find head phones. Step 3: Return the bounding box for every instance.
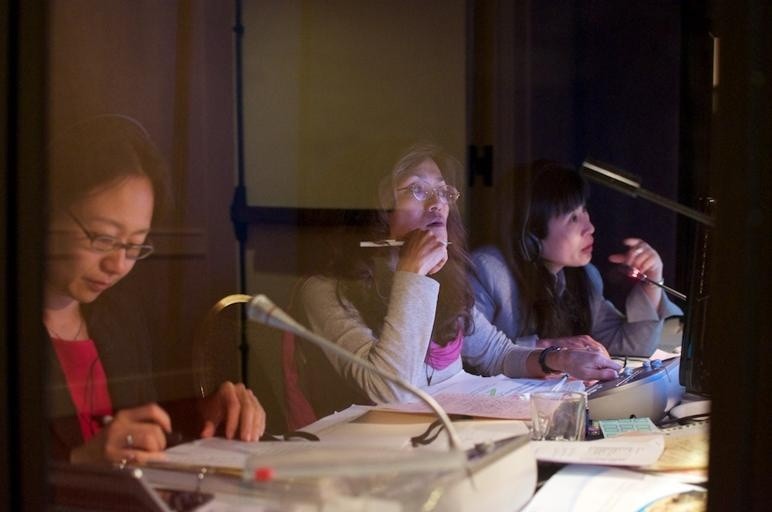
[518,195,543,263]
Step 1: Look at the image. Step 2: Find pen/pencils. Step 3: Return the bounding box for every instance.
[91,413,182,446]
[359,239,453,248]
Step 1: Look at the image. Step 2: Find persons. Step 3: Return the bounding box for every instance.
[37,115,266,479]
[460,176,686,362]
[282,143,621,432]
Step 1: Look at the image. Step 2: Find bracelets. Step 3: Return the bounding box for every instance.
[539,345,559,373]
[644,279,664,289]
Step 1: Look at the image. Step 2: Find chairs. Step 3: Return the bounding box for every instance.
[280,254,354,434]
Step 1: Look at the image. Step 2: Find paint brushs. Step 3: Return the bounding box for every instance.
[148,460,274,483]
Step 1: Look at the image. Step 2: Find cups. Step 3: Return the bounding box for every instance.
[529,391,585,441]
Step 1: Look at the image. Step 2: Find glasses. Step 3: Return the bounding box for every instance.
[394,182,462,204]
[66,208,154,260]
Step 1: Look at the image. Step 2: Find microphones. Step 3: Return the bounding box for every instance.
[620,265,688,305]
[244,292,464,455]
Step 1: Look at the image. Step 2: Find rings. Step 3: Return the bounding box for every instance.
[632,248,643,257]
[125,433,133,447]
